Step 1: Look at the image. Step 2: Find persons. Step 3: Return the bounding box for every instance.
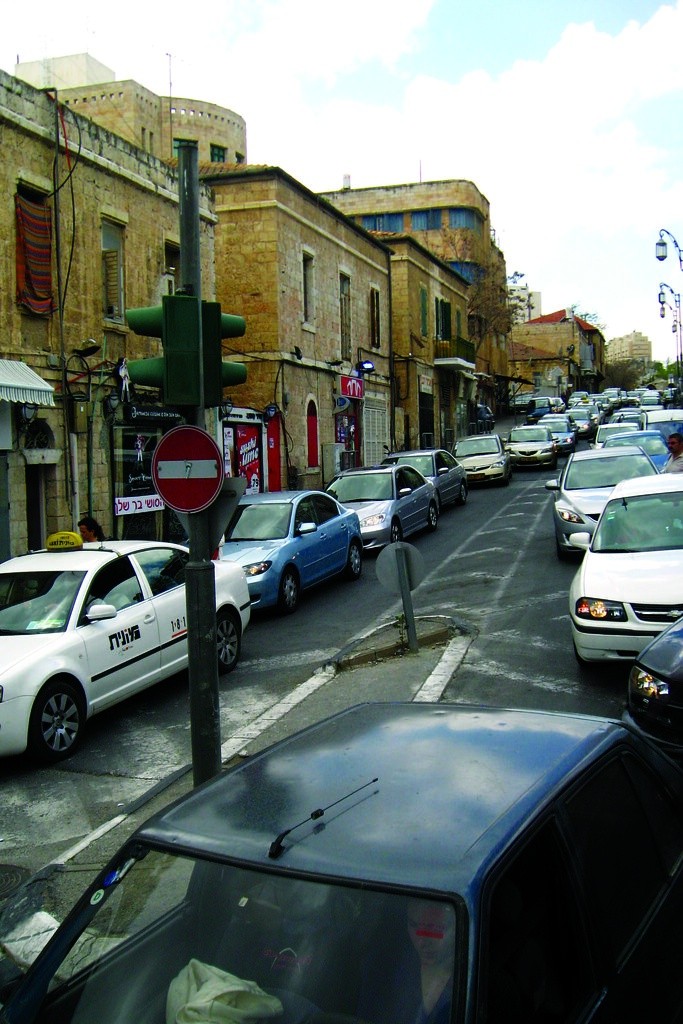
[76,517,117,543]
[474,395,480,406]
[660,433,683,474]
[560,390,573,411]
[335,895,462,1024]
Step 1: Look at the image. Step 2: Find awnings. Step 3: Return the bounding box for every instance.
[0,359,55,407]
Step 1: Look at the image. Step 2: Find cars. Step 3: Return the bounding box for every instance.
[601,429,672,471]
[587,423,640,450]
[639,410,683,439]
[380,449,468,507]
[544,445,660,563]
[451,434,512,488]
[623,616,682,768]
[537,419,578,455]
[502,424,559,471]
[178,490,365,617]
[525,387,678,439]
[323,465,440,550]
[477,404,496,432]
[569,473,683,678]
[0,532,252,767]
[0,699,683,1024]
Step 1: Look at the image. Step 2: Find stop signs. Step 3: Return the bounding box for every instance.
[151,425,224,514]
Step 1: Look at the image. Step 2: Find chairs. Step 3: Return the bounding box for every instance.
[204,872,330,997]
[347,888,429,1024]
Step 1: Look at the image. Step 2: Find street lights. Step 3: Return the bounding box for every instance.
[659,282,683,378]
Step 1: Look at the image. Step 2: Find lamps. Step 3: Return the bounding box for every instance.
[324,359,346,368]
[354,360,375,372]
[289,345,304,360]
[71,338,102,358]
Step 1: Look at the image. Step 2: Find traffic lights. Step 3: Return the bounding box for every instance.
[202,300,247,408]
[125,295,203,410]
